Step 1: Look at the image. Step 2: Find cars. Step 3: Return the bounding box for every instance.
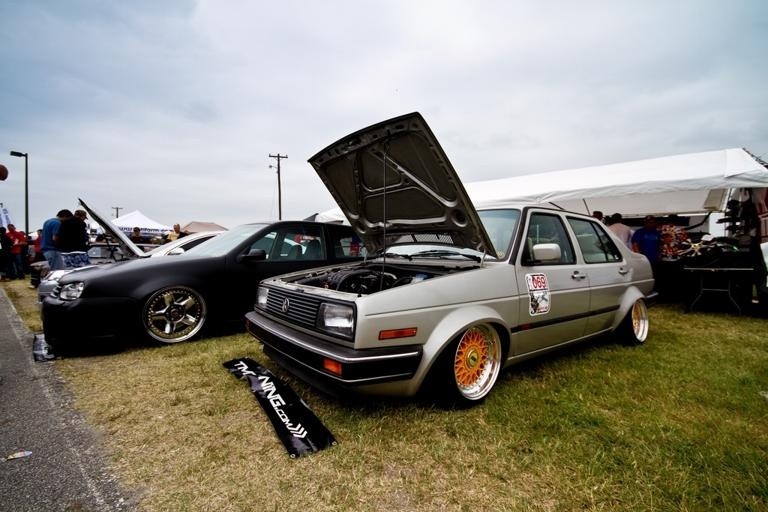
[246,106,655,409]
[28,198,309,308]
[43,220,376,352]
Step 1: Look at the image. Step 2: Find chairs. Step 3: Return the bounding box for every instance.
[528,235,613,262]
[288,239,321,258]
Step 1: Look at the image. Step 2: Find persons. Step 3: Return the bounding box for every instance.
[128,226,149,244]
[55,210,91,268]
[0,226,14,281]
[631,215,662,260]
[607,213,631,249]
[40,208,73,269]
[6,223,28,279]
[34,229,46,261]
[591,210,603,220]
[168,224,188,242]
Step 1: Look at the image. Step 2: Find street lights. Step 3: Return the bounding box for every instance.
[11,151,31,245]
